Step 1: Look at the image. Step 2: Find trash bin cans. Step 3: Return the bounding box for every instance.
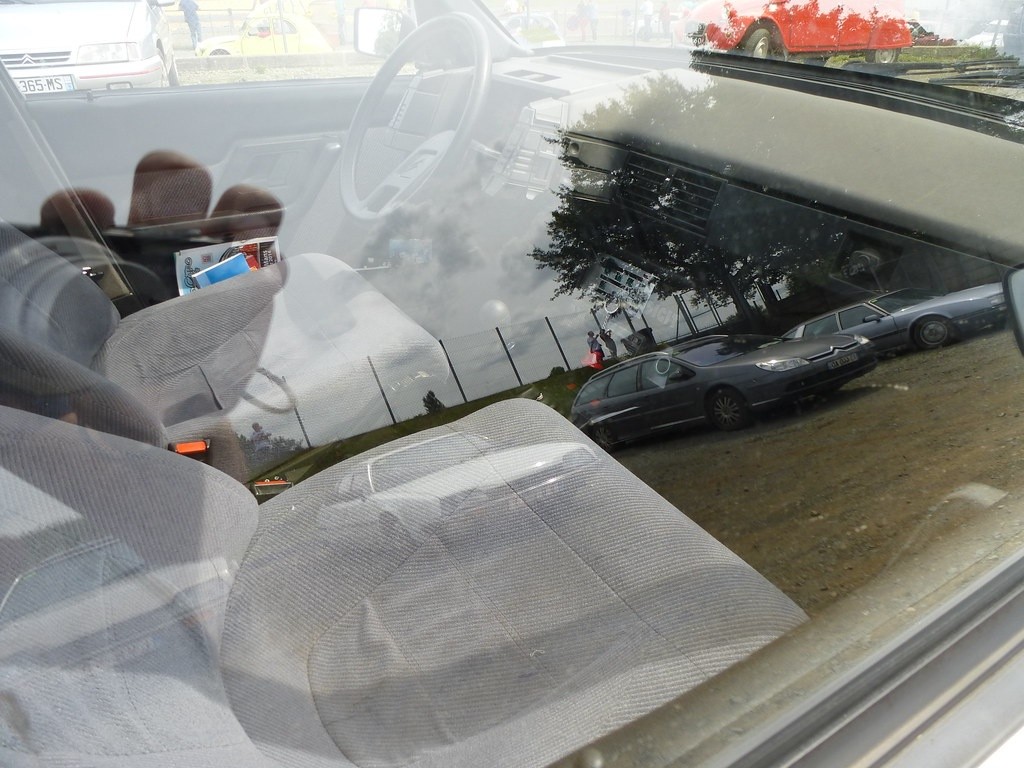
[620,327,658,358]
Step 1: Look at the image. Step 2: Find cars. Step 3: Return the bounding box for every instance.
[311,431,602,568]
[0,0,184,92]
[906,18,960,47]
[191,9,329,58]
[963,19,1010,49]
[774,279,1008,359]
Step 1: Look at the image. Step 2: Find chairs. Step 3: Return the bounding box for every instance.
[0,214,813,768]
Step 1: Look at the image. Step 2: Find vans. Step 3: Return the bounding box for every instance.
[672,0,917,67]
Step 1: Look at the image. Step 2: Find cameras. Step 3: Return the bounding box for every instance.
[0,222,274,423]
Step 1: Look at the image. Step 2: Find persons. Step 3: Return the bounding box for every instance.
[28,150,283,488]
[179,0,203,52]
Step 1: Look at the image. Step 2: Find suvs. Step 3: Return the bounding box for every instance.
[563,332,880,458]
